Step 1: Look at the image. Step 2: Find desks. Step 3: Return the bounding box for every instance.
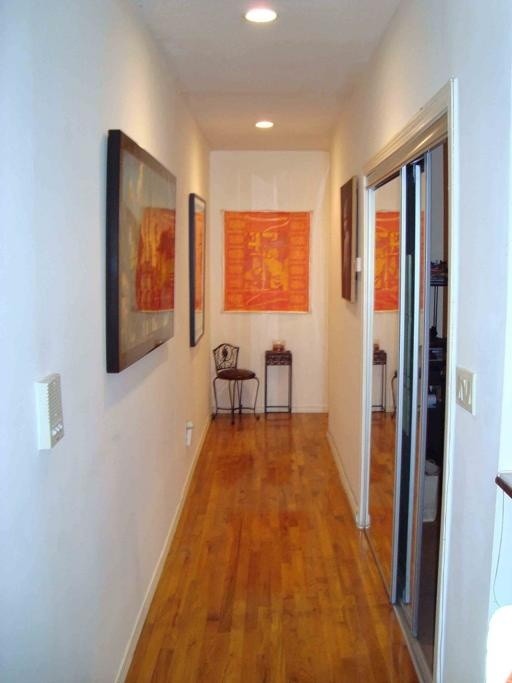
[265,349,292,416]
[372,348,386,424]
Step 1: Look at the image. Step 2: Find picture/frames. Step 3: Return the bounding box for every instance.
[108,129,176,373]
[338,175,356,304]
[189,191,207,347]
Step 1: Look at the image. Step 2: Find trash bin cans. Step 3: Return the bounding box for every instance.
[422,460,440,522]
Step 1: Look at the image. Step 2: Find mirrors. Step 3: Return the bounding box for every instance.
[365,150,427,636]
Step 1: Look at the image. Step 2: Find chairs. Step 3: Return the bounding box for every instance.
[210,342,260,425]
